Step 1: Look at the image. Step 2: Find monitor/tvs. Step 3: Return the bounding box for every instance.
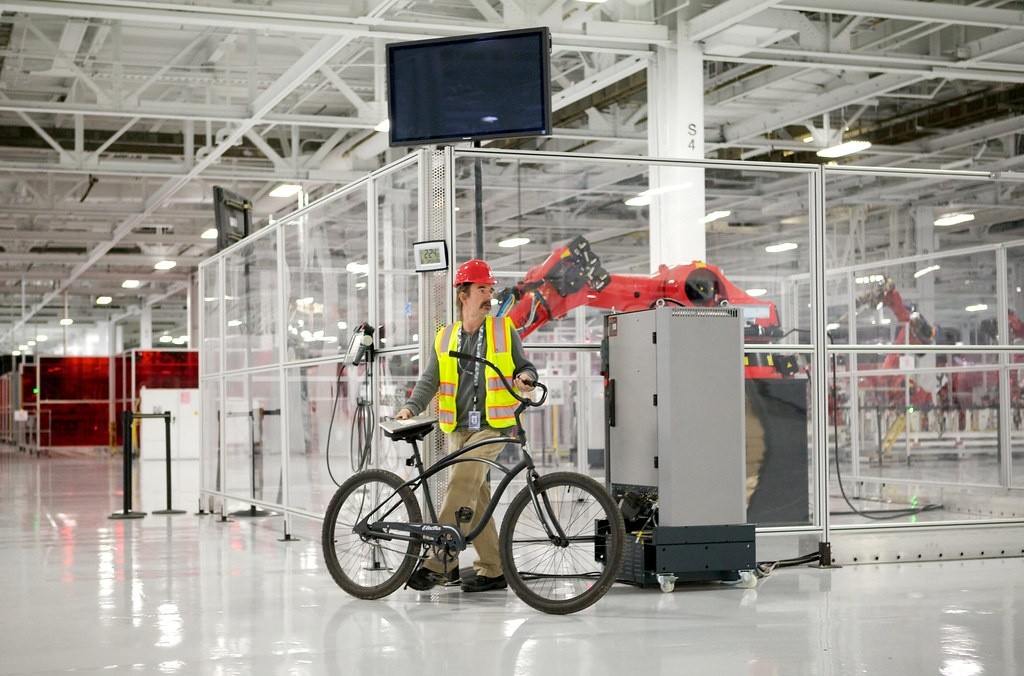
[386,27,552,147]
[213,186,253,252]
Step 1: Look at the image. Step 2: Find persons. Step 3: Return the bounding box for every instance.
[390,258,540,589]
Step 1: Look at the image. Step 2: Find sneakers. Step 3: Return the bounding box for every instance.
[407,566,464,591]
[460,575,507,592]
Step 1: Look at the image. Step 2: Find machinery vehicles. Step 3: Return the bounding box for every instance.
[500,235,846,431]
[830,272,1024,471]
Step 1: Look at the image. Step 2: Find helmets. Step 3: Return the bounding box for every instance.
[454,260,499,287]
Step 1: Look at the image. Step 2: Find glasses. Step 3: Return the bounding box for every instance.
[469,287,493,298]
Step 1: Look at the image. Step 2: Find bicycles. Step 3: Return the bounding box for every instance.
[321,360,631,615]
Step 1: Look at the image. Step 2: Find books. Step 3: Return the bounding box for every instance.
[377,415,438,434]
